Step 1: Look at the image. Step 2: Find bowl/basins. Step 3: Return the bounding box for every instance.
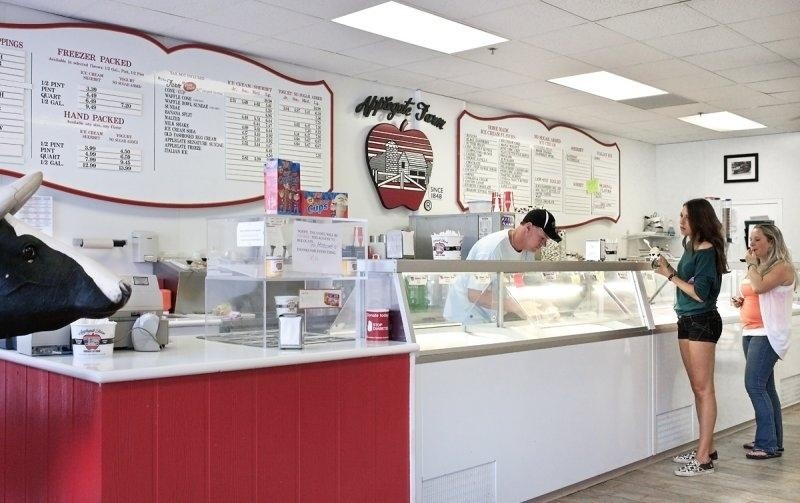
[467,201,491,213]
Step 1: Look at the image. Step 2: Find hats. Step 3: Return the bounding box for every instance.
[523,208,561,243]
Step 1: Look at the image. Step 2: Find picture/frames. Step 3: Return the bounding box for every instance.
[722,153,758,182]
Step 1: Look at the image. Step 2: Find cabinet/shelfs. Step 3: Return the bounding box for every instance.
[626,231,676,262]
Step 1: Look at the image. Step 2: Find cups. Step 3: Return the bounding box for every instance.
[273,295,298,316]
[605,242,618,262]
[430,235,463,261]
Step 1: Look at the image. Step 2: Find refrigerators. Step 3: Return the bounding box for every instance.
[408,212,515,260]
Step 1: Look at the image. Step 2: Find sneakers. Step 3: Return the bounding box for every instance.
[673,459,718,476]
[673,449,718,463]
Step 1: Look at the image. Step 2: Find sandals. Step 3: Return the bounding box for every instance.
[744,441,784,459]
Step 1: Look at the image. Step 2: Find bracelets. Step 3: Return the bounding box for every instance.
[668,274,675,281]
[667,264,670,268]
[747,263,757,271]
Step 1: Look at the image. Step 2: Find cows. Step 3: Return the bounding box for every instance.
[0,171,132,340]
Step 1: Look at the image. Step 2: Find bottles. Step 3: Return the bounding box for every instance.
[369,233,386,259]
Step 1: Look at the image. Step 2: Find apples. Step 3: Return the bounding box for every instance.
[366,119,433,211]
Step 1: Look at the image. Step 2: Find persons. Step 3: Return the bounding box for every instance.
[731,222,800,460]
[654,198,730,477]
[442,208,561,326]
